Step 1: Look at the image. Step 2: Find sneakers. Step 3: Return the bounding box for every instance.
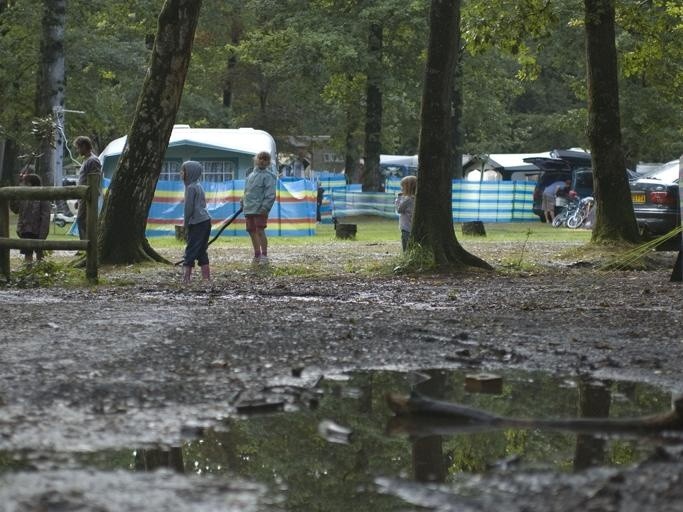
[252,255,270,267]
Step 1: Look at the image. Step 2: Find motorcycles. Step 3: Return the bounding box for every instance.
[50,198,80,227]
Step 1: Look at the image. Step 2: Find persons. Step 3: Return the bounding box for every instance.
[316,187,324,224]
[540,179,571,224]
[9,172,53,274]
[69,134,103,268]
[394,174,418,253]
[176,159,213,286]
[289,150,311,170]
[238,150,278,267]
[531,174,553,222]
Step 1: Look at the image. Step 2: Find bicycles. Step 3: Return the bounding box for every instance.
[550,193,588,228]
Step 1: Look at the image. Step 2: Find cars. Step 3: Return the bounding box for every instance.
[627,158,679,234]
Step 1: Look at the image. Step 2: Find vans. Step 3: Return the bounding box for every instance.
[521,148,642,224]
